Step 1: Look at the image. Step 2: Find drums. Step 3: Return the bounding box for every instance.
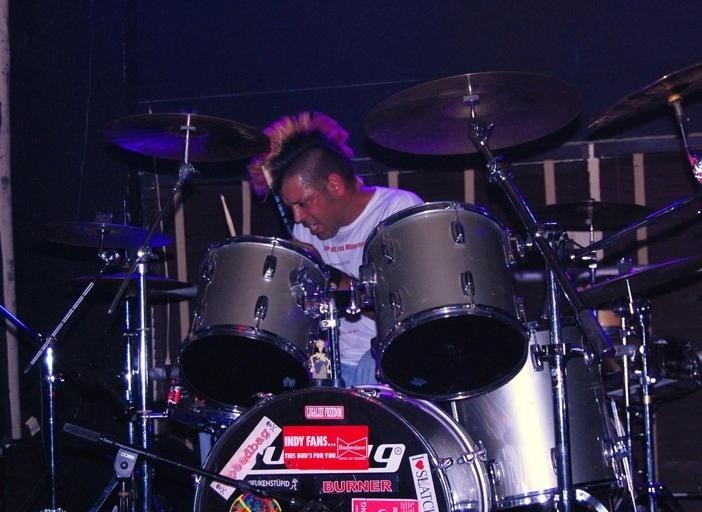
[194,385,498,512]
[445,316,620,512]
[356,201,531,401]
[602,336,701,404]
[177,235,334,414]
[166,375,245,428]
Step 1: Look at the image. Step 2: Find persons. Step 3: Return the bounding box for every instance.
[245,110,425,389]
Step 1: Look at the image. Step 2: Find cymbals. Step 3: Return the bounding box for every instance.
[563,192,700,267]
[365,71,584,154]
[515,199,653,230]
[63,274,192,293]
[589,64,702,132]
[560,256,701,313]
[36,220,176,252]
[101,114,272,162]
